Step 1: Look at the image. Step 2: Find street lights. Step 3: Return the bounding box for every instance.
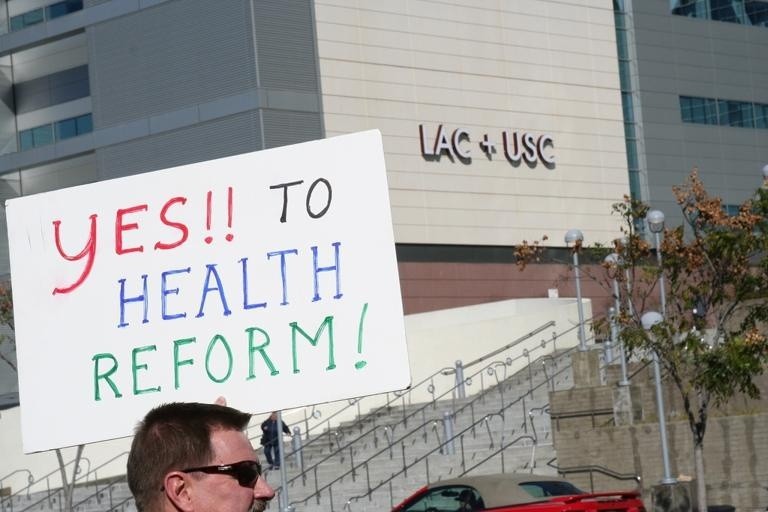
[647,209,672,317]
[639,310,678,485]
[618,234,634,320]
[605,253,637,423]
[564,226,588,347]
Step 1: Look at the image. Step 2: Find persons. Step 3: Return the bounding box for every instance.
[261,411,292,470]
[455,489,477,512]
[125,402,274,512]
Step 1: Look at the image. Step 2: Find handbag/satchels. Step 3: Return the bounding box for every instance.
[260,430,270,446]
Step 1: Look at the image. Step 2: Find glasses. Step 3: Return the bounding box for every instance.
[161,461,261,492]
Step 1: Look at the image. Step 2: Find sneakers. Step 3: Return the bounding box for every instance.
[274,464,279,470]
[268,464,273,470]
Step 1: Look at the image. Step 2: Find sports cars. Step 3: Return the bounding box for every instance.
[391,472,645,512]
